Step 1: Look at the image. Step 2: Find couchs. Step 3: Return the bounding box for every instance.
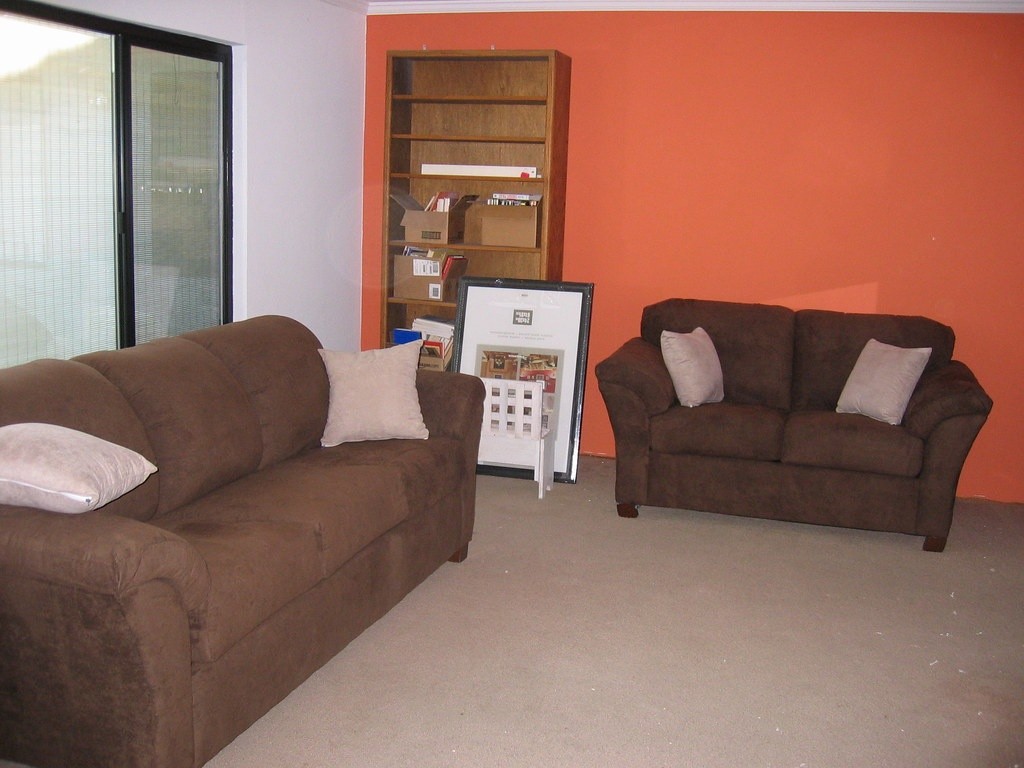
[596,296,993,554]
[0,313,487,768]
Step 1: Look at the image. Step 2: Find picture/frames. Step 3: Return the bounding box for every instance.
[446,275,595,479]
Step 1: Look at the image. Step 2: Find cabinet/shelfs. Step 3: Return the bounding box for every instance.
[379,48,572,350]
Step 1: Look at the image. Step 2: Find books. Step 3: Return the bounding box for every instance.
[389,314,455,359]
[488,194,543,207]
[403,246,468,276]
[423,191,479,211]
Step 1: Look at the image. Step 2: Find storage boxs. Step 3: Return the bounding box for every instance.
[387,333,448,372]
[466,197,542,248]
[394,253,459,300]
[390,187,480,245]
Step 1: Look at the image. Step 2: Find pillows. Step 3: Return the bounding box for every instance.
[837,337,933,426]
[659,324,723,408]
[1,422,158,514]
[317,339,430,440]
